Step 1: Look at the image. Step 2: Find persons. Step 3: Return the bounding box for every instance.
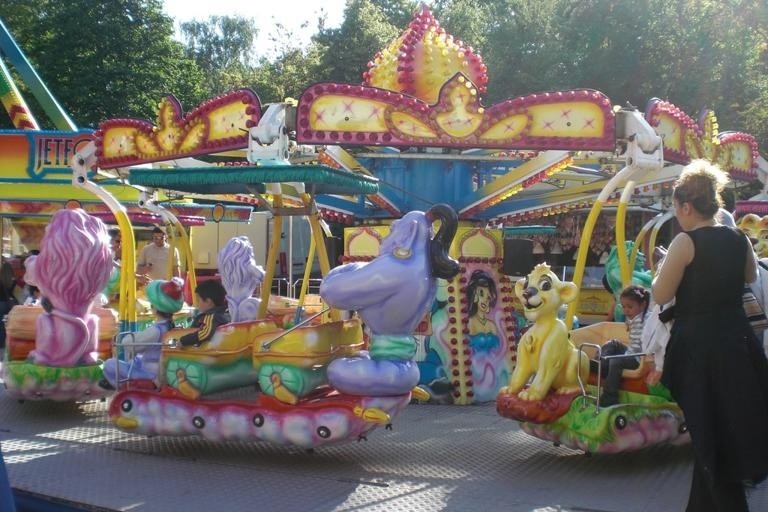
[176,279,231,347]
[108,228,151,286]
[590,284,652,406]
[136,228,181,280]
[0,256,42,347]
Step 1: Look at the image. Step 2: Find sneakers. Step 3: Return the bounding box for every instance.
[595,391,620,408]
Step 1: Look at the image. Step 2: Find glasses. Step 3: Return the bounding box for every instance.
[153,236,165,240]
